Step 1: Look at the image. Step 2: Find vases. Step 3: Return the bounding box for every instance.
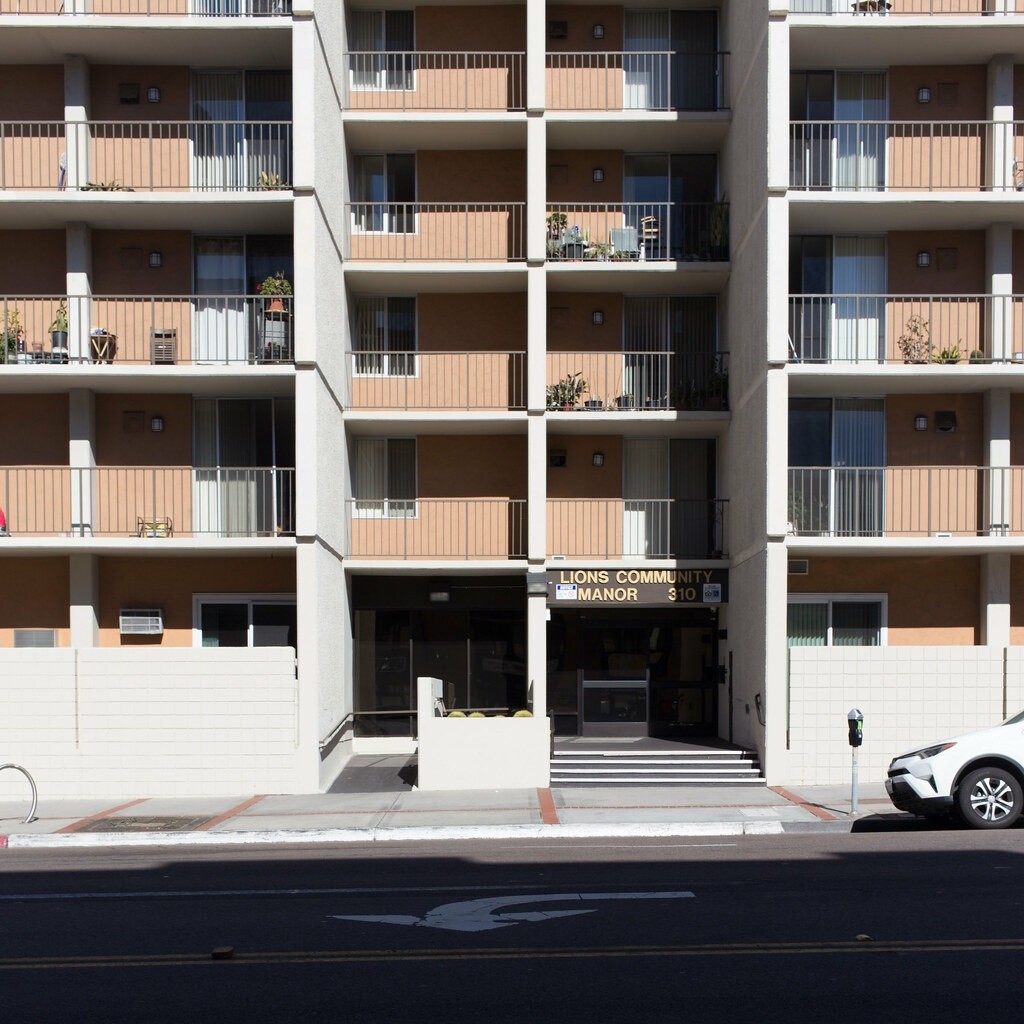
[563,242,588,262]
[270,349,287,361]
[31,340,46,353]
[645,400,666,411]
[615,396,638,411]
[584,400,602,411]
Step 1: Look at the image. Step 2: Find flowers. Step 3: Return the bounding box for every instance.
[563,226,585,245]
[267,341,286,348]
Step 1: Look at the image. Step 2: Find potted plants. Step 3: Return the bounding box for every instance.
[546,213,569,241]
[1,308,23,363]
[549,370,587,411]
[259,276,292,313]
[545,240,563,262]
[48,298,70,350]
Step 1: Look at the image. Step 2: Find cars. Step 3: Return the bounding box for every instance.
[884,710,1024,829]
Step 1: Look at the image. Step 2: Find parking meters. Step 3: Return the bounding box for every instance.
[848,709,864,815]
